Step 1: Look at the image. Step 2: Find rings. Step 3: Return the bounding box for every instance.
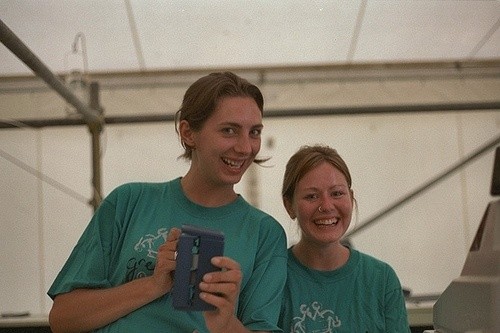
[174,251,177,260]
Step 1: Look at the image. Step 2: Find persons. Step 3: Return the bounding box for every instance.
[47,72,288,333]
[277,145,412,333]
